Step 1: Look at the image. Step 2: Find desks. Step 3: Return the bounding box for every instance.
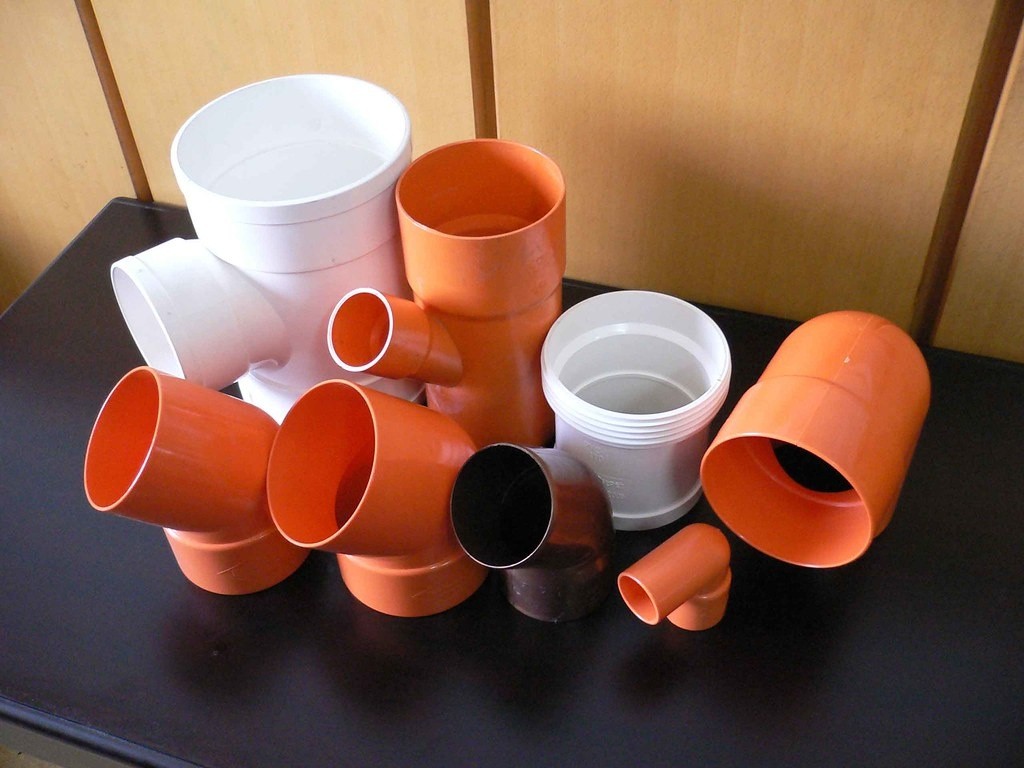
[0,194,1024,767]
